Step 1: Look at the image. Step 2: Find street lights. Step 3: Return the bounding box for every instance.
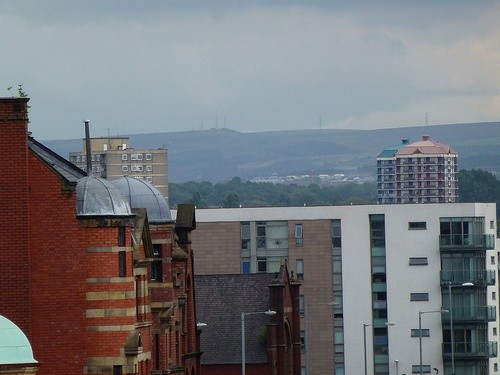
[241,310,277,375]
[363,322,396,375]
[419,310,450,375]
[447,281,474,375]
